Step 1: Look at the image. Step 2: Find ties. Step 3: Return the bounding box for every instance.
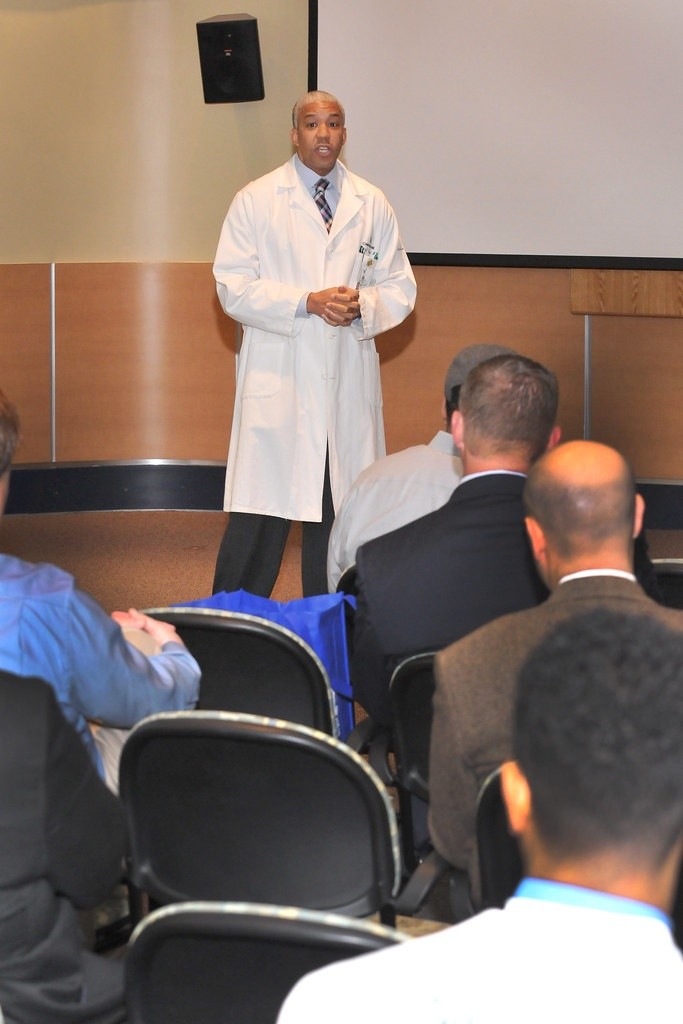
[312,179,333,234]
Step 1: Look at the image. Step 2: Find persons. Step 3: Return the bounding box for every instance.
[1,344,683,1023]
[211,93,419,599]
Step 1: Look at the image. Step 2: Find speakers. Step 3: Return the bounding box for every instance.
[195,14,265,104]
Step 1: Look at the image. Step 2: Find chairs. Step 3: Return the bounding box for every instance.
[110,558,683,1022]
